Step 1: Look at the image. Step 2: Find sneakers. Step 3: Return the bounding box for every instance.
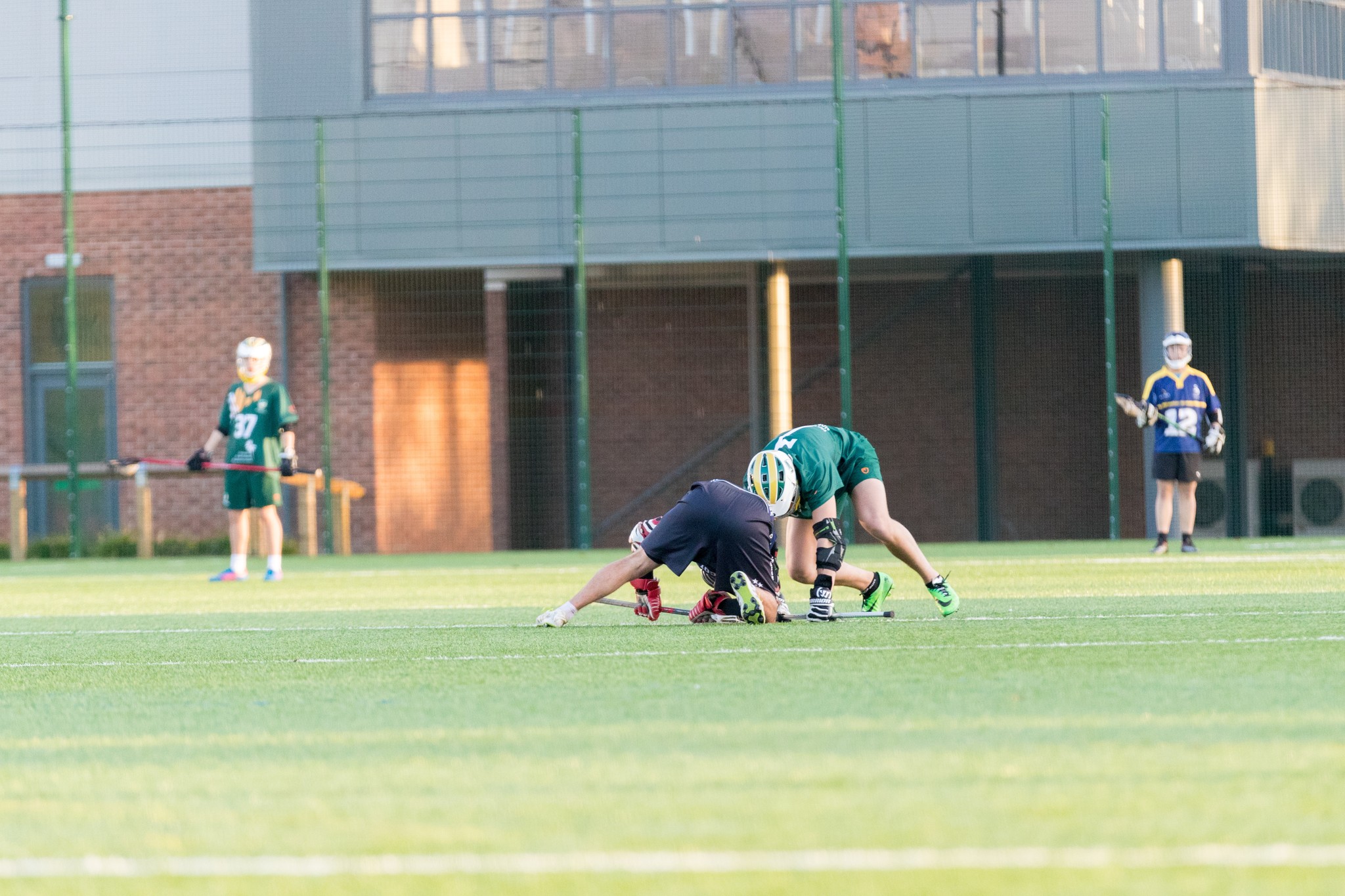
[860,571,895,613]
[927,571,960,616]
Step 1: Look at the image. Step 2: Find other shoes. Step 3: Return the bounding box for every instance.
[206,567,250,583]
[730,570,766,625]
[263,568,284,582]
[535,607,565,628]
[1181,539,1197,553]
[1150,541,1169,555]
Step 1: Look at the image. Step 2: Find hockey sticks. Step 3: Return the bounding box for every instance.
[1113,392,1206,445]
[106,456,321,476]
[775,611,895,621]
[594,598,692,615]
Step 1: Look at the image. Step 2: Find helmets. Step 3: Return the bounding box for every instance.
[747,449,800,519]
[1163,330,1193,370]
[236,336,272,384]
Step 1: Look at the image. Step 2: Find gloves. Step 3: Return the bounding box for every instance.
[1201,423,1227,456]
[806,587,834,622]
[1137,400,1159,427]
[775,593,791,623]
[185,448,212,473]
[279,452,296,476]
[688,589,732,623]
[630,577,662,621]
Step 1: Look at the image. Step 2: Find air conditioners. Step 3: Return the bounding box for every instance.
[1192,458,1261,537]
[1293,459,1345,537]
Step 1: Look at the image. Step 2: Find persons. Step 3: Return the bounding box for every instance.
[742,425,960,620]
[534,480,793,625]
[186,337,301,584]
[1133,331,1225,552]
